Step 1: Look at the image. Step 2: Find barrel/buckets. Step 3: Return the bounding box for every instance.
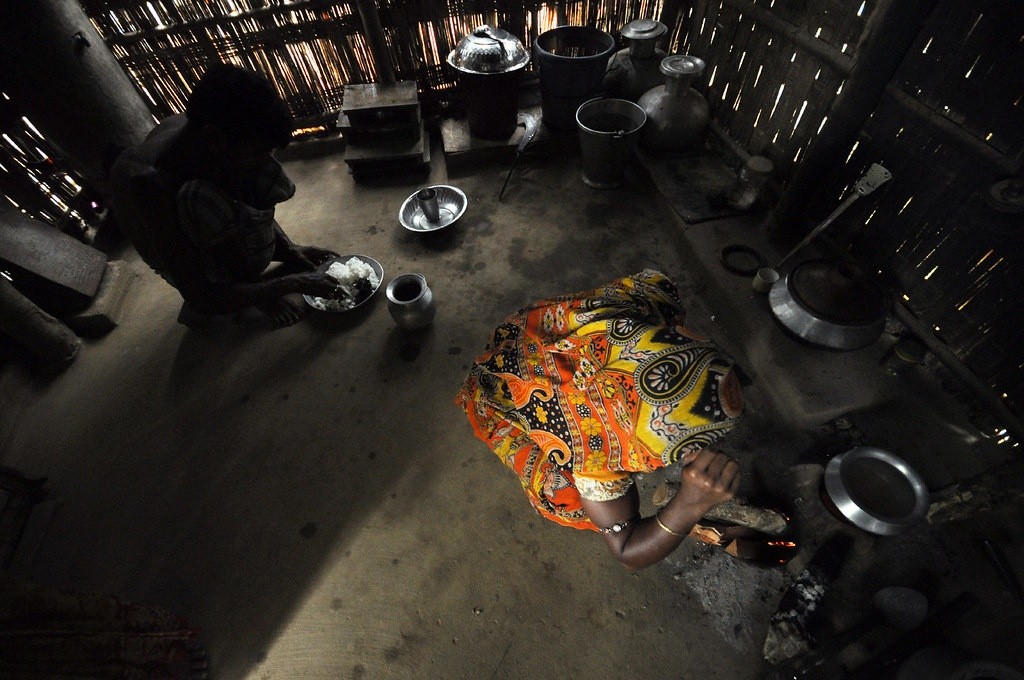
[575,97,646,189]
[533,25,615,130]
[458,68,523,140]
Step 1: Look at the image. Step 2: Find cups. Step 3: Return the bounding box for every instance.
[416,188,440,222]
[753,269,778,292]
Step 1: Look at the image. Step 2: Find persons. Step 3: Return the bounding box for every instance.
[453,269,746,571]
[107,63,346,329]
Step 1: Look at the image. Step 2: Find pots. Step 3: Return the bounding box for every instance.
[821,446,928,537]
[769,259,890,351]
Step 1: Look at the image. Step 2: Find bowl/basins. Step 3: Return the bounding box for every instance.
[303,255,384,313]
[446,29,530,74]
[399,185,468,232]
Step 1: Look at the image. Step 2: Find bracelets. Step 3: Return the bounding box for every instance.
[656,507,691,536]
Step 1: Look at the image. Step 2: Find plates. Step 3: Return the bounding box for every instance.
[720,243,762,274]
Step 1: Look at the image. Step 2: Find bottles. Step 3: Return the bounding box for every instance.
[604,20,668,103]
[881,335,927,378]
[386,273,436,331]
[636,55,710,155]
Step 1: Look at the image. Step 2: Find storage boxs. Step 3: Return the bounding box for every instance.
[337,81,431,183]
